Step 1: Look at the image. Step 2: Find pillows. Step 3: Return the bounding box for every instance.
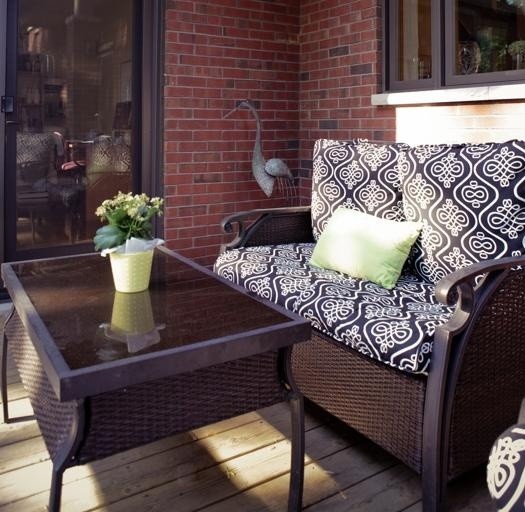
[16,132,53,192]
[87,133,133,174]
[486,397,525,511]
[311,139,414,277]
[398,139,525,287]
[309,205,421,291]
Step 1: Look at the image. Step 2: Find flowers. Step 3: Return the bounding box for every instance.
[94,190,165,252]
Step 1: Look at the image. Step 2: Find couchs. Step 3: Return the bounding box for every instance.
[212,204,524,512]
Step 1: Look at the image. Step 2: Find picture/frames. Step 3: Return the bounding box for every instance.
[112,100,134,128]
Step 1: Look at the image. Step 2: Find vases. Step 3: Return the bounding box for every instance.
[109,293,155,335]
[109,254,153,294]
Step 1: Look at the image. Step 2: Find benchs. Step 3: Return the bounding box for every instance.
[31,173,133,239]
